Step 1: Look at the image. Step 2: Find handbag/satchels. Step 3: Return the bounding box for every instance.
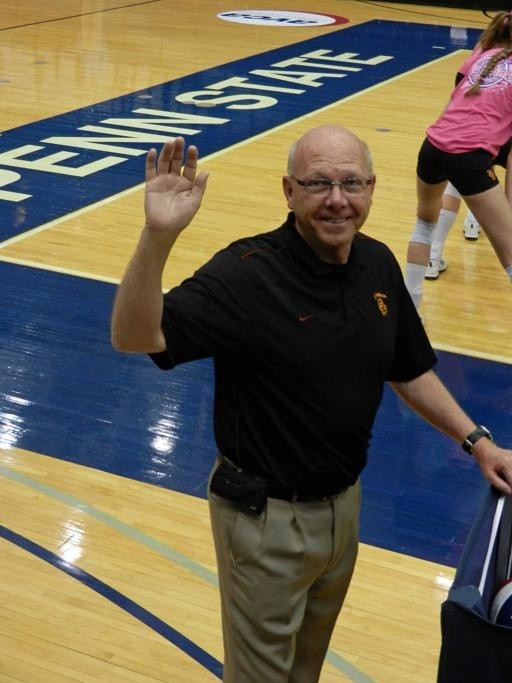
[210,464,266,517]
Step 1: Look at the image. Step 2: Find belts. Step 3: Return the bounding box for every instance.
[266,486,324,502]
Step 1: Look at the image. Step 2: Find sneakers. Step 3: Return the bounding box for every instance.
[464,220,480,238]
[424,257,446,279]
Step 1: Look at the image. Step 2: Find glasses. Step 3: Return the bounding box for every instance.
[291,176,372,193]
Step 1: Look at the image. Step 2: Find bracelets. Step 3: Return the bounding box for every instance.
[462,425,493,455]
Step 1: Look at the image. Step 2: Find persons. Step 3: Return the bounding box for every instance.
[462,134,510,241]
[402,8,510,307]
[422,8,512,281]
[109,122,510,681]
